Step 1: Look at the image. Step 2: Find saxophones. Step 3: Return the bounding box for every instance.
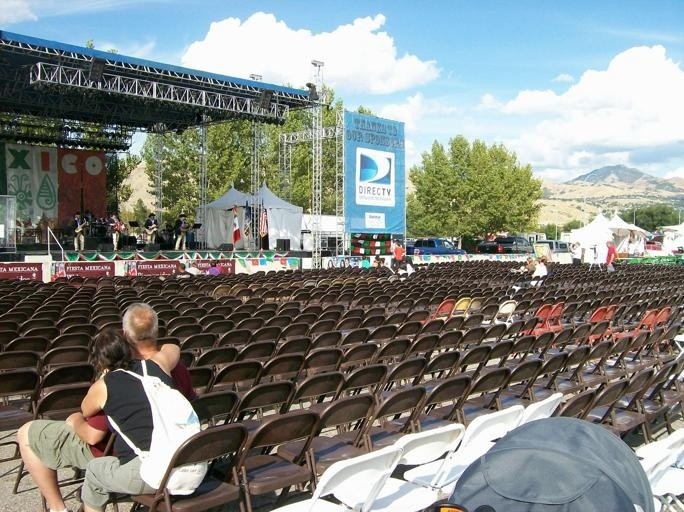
[75,216,87,232]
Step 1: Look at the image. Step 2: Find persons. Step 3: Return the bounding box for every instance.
[606,241,617,268]
[519,264,535,288]
[173,214,189,251]
[511,256,533,274]
[204,261,221,277]
[359,256,371,269]
[67,209,85,252]
[612,232,620,246]
[530,254,548,289]
[392,244,405,270]
[628,240,635,258]
[144,213,157,246]
[569,241,582,265]
[105,211,121,251]
[77,323,209,512]
[172,263,192,279]
[389,239,399,259]
[344,258,352,268]
[16,301,198,512]
[327,259,335,269]
[338,259,345,268]
[184,261,202,275]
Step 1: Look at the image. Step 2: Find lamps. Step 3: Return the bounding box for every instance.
[306,82,319,101]
[251,89,273,108]
[87,56,105,81]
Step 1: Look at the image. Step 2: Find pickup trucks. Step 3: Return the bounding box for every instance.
[406,237,467,255]
[480,237,534,253]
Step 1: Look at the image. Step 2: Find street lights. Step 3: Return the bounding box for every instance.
[634,208,638,225]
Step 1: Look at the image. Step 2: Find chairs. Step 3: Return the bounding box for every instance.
[0,260,684,512]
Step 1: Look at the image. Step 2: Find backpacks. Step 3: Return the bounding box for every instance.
[101,359,208,497]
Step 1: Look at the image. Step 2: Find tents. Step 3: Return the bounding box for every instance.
[442,413,657,511]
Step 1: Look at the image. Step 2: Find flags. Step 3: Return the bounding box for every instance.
[230,205,241,244]
[257,205,268,238]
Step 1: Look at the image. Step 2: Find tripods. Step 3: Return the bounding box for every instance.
[190,228,202,251]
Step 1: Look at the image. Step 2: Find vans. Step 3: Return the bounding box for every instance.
[535,240,572,253]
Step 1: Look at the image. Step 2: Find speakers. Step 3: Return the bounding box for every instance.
[97,243,113,252]
[217,244,233,251]
[277,239,290,251]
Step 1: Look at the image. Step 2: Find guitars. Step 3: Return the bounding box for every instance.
[146,221,165,234]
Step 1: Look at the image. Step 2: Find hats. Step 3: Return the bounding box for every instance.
[178,214,187,218]
[149,213,156,218]
